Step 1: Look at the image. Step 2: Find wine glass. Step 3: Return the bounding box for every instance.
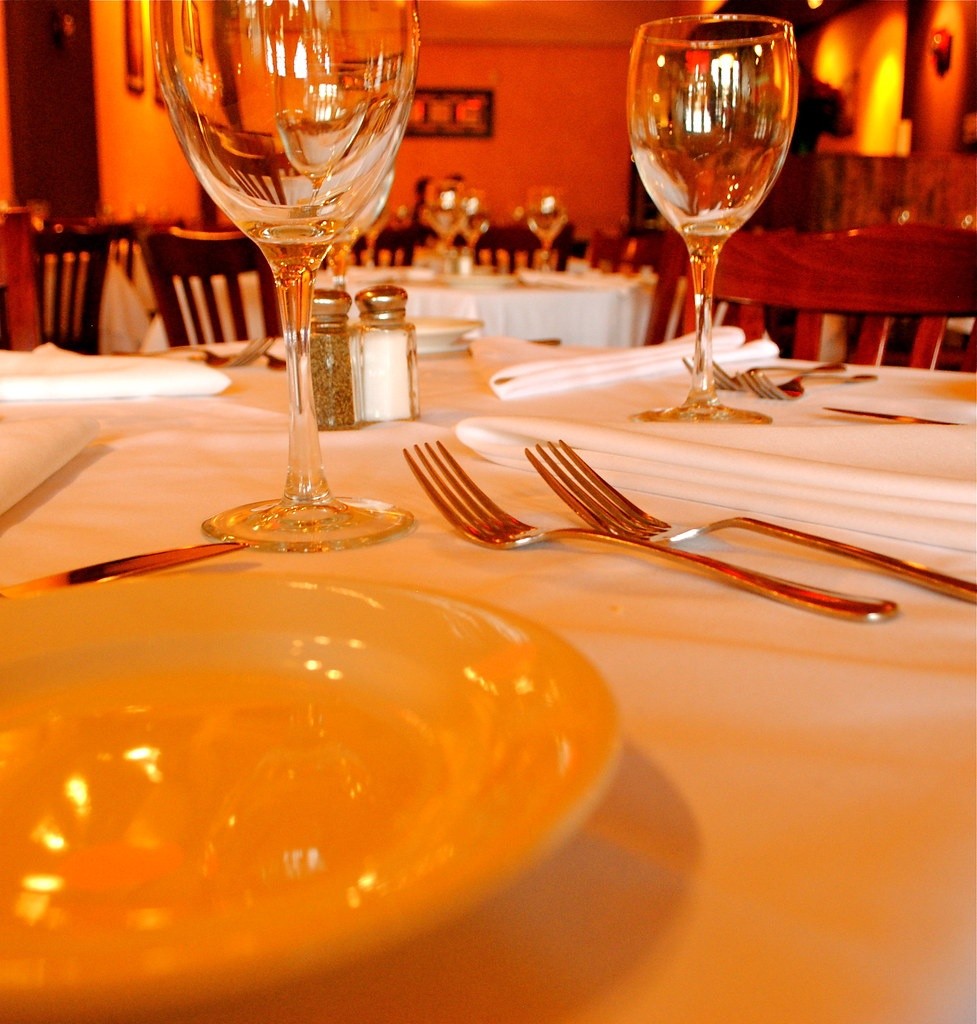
[625,11,797,429]
[427,178,461,279]
[148,1,417,553]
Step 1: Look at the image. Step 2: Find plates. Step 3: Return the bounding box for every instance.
[413,341,475,356]
[390,317,483,345]
[0,556,628,1024]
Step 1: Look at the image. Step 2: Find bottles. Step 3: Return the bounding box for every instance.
[309,293,356,431]
[349,285,420,426]
[523,184,569,278]
[456,186,492,278]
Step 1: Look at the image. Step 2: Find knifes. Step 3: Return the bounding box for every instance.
[825,406,961,428]
[0,539,247,602]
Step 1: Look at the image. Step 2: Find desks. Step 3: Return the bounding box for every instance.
[138,267,654,359]
[0,341,977,1024]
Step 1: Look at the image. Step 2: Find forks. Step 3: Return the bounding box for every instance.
[526,440,977,608]
[733,369,878,402]
[110,335,279,369]
[679,351,845,392]
[400,438,904,626]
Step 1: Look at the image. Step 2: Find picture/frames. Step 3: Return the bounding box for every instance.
[181,0,204,60]
[123,0,145,91]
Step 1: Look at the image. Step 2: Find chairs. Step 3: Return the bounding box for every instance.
[0,203,977,374]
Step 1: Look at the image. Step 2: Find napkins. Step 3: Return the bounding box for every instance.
[468,326,779,400]
[0,344,233,398]
